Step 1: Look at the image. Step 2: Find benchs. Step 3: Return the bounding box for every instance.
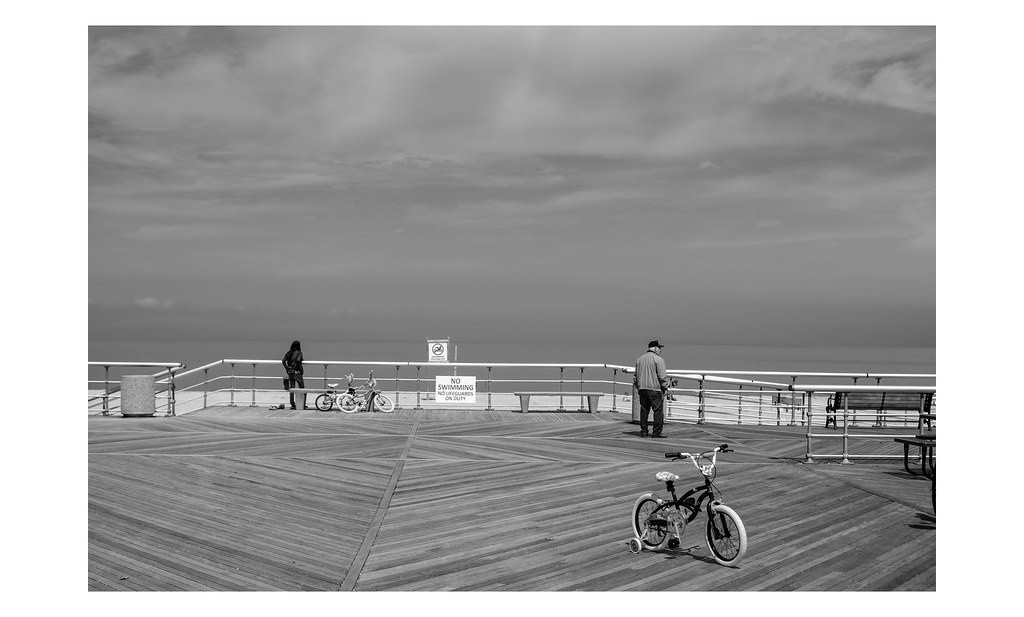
[772,395,802,412]
[825,391,935,431]
[514,391,604,414]
[894,434,936,475]
[289,388,381,410]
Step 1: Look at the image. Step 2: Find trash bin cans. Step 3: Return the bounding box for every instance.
[120,375,156,417]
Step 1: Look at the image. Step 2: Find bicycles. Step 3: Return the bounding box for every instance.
[315,373,363,412]
[627,443,748,567]
[335,370,395,413]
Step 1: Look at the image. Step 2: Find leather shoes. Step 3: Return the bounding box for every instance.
[651,432,667,438]
[639,430,648,438]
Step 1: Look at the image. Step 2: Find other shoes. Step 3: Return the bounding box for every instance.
[291,405,296,409]
[304,405,308,408]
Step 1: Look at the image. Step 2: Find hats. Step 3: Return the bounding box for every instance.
[647,340,664,348]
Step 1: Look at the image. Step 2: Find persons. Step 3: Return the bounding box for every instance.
[282,340,308,409]
[633,340,668,438]
